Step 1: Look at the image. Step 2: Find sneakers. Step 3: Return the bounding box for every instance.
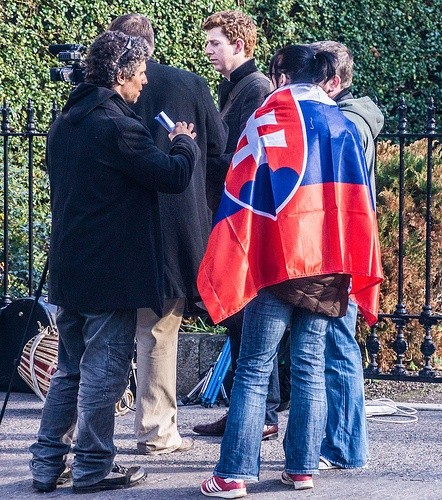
[281,470,314,490]
[200,476,247,499]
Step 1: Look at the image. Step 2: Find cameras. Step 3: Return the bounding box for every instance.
[49,43,86,86]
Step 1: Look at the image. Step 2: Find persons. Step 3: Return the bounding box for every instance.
[308,41,384,470]
[193,11,292,441]
[30,31,200,493]
[196,44,384,499]
[72,13,229,455]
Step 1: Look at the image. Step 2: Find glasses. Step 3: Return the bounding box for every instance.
[266,73,290,80]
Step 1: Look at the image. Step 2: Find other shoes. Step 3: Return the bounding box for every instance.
[318,456,340,470]
[71,463,148,493]
[33,467,73,492]
[142,436,194,455]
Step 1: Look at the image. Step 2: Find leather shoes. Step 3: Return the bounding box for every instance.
[261,423,278,441]
[192,414,228,435]
